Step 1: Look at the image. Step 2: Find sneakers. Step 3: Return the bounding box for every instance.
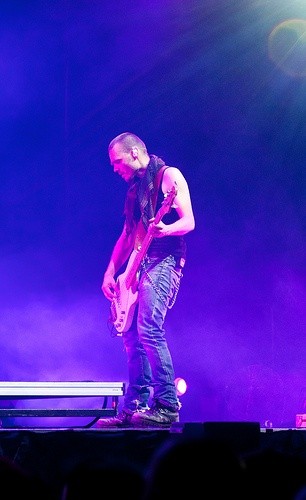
[132,410,179,427]
[97,417,129,428]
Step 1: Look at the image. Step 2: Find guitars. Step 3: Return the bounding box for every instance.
[110,185,178,333]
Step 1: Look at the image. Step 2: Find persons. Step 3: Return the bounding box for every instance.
[97,132,195,428]
